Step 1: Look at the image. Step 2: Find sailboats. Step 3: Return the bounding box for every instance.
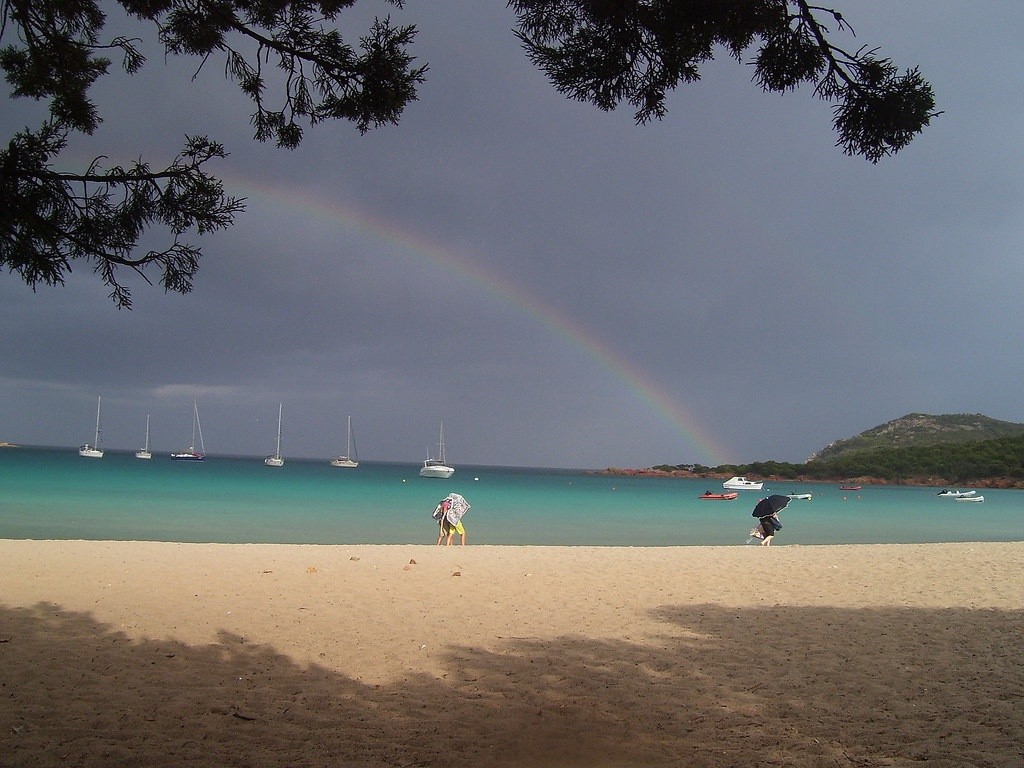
[419,419,455,479]
[170,401,206,461]
[330,416,359,468]
[135,415,152,458]
[78,396,106,458]
[264,402,285,466]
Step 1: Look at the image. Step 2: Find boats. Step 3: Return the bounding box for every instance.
[698,493,738,500]
[0,443,20,448]
[937,490,976,498]
[955,496,985,502]
[722,477,763,490]
[787,494,812,499]
[840,486,862,490]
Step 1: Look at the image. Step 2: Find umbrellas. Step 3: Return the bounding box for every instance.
[752,495,792,521]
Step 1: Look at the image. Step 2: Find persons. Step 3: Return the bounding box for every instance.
[960,495,965,498]
[705,490,711,495]
[760,514,777,546]
[433,492,469,545]
[938,489,948,495]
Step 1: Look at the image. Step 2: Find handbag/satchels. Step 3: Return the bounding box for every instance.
[750,523,765,540]
[769,515,782,532]
